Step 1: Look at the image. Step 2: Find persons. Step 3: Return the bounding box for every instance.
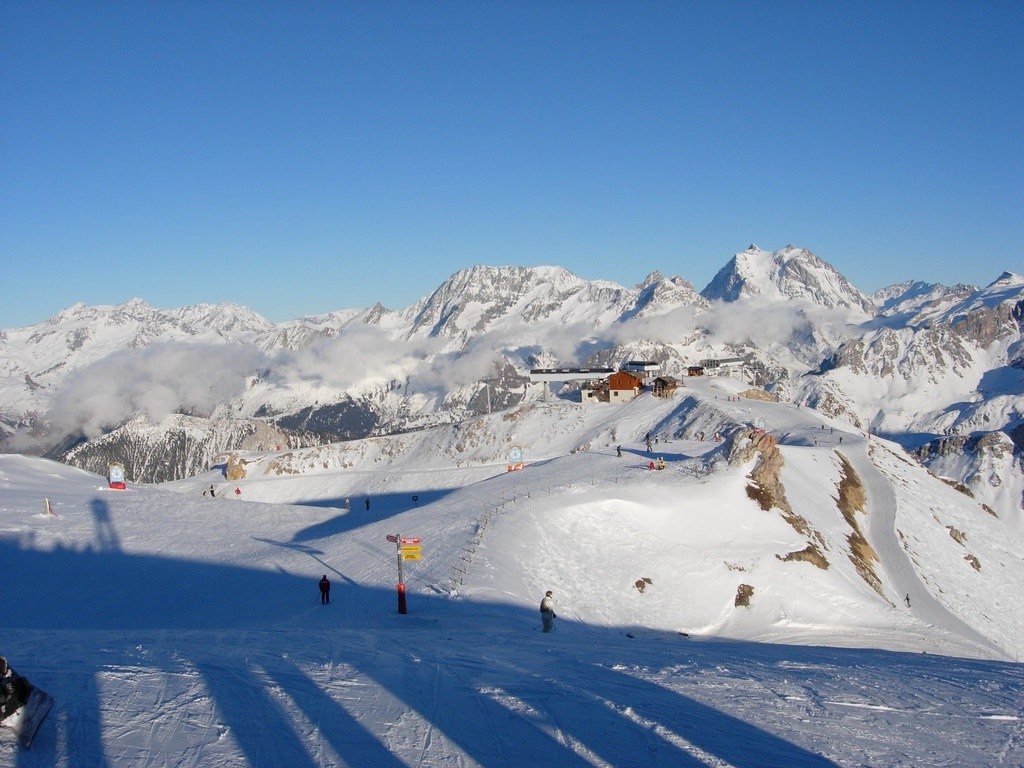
[649,461,654,471]
[210,484,216,497]
[365,499,369,510]
[904,593,911,607]
[616,445,622,457]
[714,396,740,402]
[796,403,801,410]
[0,657,30,721]
[646,439,652,452]
[831,428,833,434]
[319,575,330,604]
[713,431,721,442]
[235,487,241,499]
[647,432,649,439]
[822,425,824,429]
[839,437,844,444]
[541,590,556,633]
[345,497,350,509]
[700,432,704,441]
[654,433,658,444]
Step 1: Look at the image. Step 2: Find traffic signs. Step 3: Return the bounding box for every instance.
[386,535,397,542]
[400,537,421,544]
[399,546,422,552]
[401,553,421,560]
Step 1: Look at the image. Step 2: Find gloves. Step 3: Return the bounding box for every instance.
[553,614,556,618]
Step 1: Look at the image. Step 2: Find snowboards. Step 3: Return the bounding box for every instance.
[0,655,58,749]
